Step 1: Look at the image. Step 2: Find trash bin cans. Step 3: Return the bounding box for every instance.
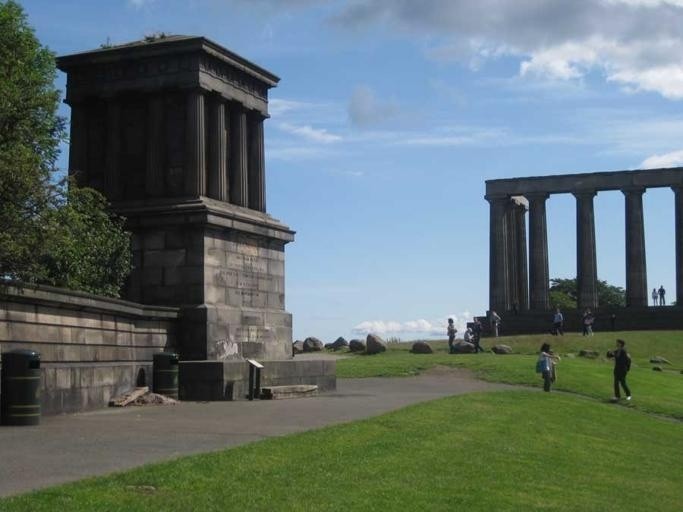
[153,353,179,401]
[2,348,41,426]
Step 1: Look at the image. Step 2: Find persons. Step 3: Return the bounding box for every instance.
[651,288,658,307]
[446,317,457,355]
[470,317,483,354]
[658,285,665,306]
[605,337,632,401]
[552,308,563,336]
[582,306,595,336]
[463,328,474,343]
[488,310,501,338]
[536,343,561,392]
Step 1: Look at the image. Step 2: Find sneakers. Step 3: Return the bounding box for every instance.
[612,396,632,401]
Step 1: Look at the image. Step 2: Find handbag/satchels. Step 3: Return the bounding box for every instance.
[536,359,550,373]
